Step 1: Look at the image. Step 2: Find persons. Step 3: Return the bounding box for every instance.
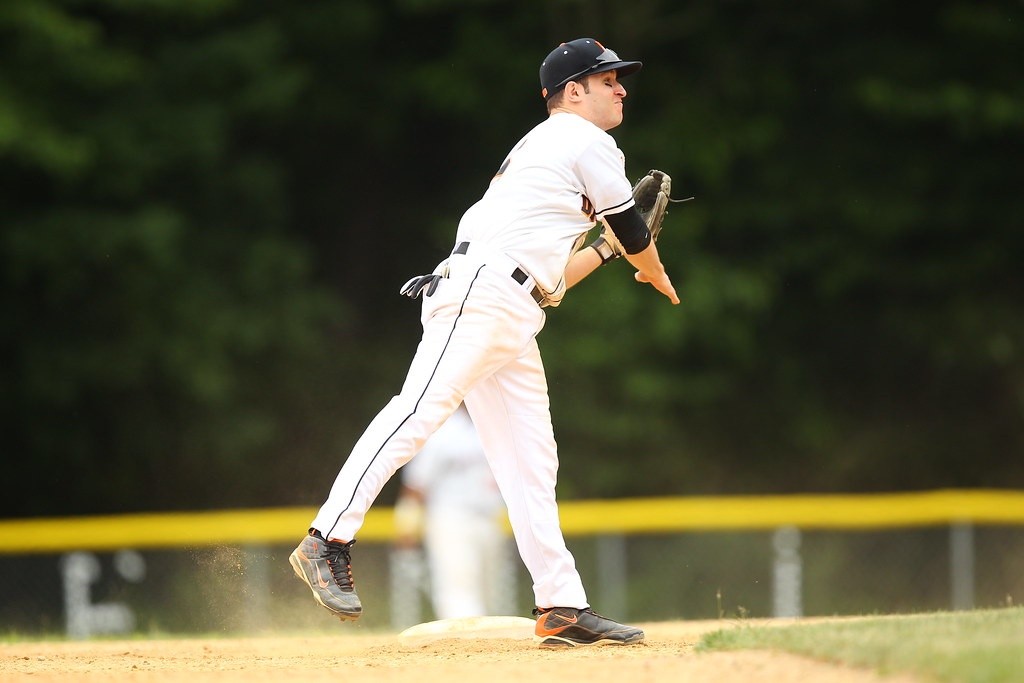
[288,39,680,651]
[399,403,508,619]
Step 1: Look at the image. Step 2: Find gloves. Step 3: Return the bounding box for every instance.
[399,266,450,299]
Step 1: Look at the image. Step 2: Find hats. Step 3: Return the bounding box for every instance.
[539,37,642,103]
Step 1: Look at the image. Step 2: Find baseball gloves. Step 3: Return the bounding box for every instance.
[602,169,670,255]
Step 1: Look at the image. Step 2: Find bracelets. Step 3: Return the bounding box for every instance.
[590,237,616,265]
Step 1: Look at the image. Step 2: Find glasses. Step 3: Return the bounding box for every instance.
[554,48,622,88]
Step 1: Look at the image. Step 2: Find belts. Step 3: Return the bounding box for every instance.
[452,241,550,309]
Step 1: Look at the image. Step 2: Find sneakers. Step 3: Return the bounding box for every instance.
[531,607,644,650]
[288,529,363,622]
